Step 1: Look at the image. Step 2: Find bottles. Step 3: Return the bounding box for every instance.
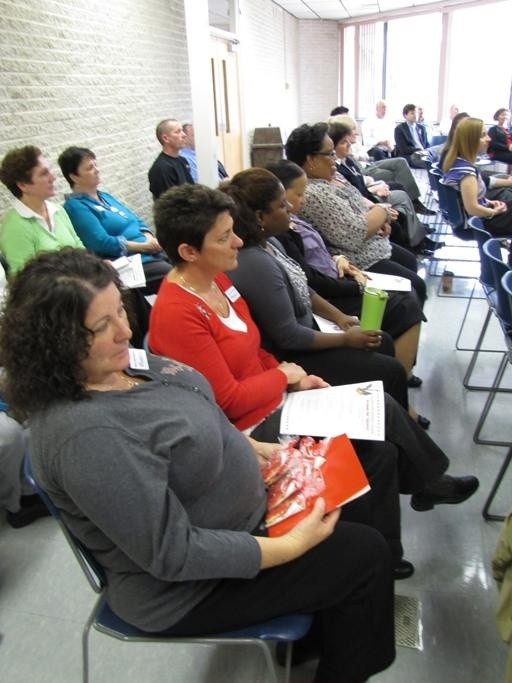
[360,287,390,330]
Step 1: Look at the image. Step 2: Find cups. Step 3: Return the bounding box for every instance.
[442,271,454,294]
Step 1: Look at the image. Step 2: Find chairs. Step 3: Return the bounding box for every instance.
[22,451,315,683]
[428,163,512,521]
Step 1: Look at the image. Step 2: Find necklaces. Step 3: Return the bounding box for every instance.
[86,373,139,390]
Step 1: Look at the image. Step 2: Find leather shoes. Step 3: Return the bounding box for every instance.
[411,475,479,511]
[7,493,52,528]
[392,560,414,580]
[412,200,445,256]
[407,375,421,387]
[417,415,431,430]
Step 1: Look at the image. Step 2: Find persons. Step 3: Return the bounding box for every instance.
[148,183,480,577]
[0,246,396,683]
[0,256,34,528]
[360,101,462,168]
[263,106,444,428]
[0,146,151,347]
[58,146,173,295]
[438,108,512,237]
[148,117,197,199]
[220,167,409,413]
